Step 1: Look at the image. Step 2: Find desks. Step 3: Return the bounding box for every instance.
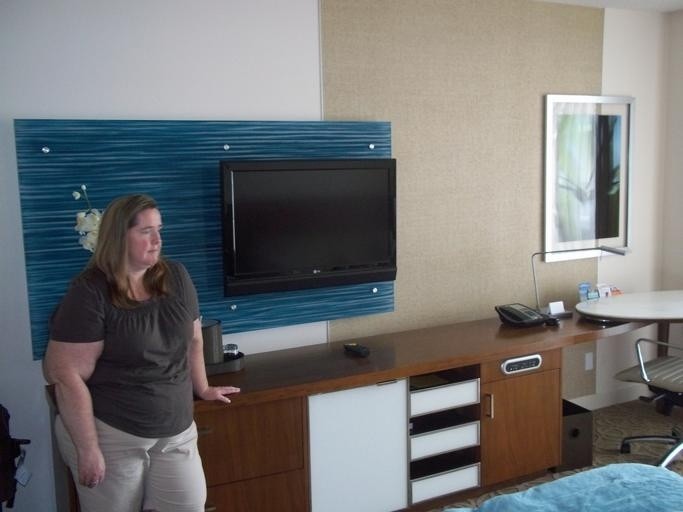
[574,288,681,357]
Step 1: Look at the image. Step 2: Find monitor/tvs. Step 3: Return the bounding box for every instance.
[219,156,399,299]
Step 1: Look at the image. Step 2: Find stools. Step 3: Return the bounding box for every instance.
[614,336,682,469]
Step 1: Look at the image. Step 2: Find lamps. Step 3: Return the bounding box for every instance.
[529,245,628,318]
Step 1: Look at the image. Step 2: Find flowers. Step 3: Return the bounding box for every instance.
[73,183,103,252]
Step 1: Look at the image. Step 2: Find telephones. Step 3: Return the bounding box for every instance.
[495,303,550,327]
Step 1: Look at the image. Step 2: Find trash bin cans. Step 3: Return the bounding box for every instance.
[562,399,593,470]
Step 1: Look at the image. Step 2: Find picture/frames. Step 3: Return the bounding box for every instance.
[541,92,637,262]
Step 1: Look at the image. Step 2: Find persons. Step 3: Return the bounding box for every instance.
[44,194,242,512]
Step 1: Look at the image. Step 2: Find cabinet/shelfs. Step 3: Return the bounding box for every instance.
[44,312,651,511]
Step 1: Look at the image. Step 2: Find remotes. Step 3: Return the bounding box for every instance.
[343,340,371,358]
